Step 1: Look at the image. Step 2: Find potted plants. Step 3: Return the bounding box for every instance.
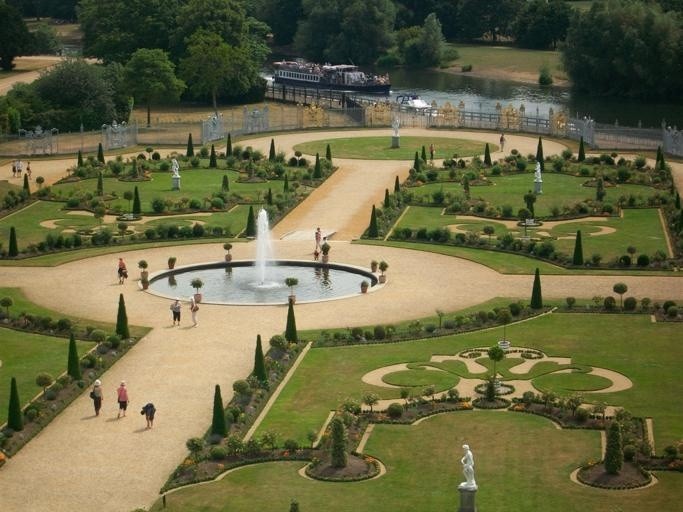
[494,309,513,350]
[487,345,504,391]
[517,208,532,241]
[122,191,134,220]
[137,243,388,305]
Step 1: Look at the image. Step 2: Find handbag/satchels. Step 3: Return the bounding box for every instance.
[90,392,95,399]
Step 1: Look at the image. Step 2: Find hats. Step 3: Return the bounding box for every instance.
[120,381,126,386]
[94,380,101,386]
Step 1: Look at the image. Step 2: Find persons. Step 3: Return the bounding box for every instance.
[532,160,542,183]
[459,444,476,487]
[139,402,156,430]
[390,119,400,137]
[319,237,328,246]
[187,295,199,328]
[428,144,435,161]
[88,378,103,416]
[169,159,181,178]
[168,297,184,326]
[311,248,320,261]
[24,161,33,180]
[10,159,16,177]
[115,381,129,418]
[499,132,506,152]
[313,226,321,251]
[116,256,128,284]
[15,159,23,178]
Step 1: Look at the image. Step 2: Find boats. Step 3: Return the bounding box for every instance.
[388,93,443,115]
[271,53,399,96]
